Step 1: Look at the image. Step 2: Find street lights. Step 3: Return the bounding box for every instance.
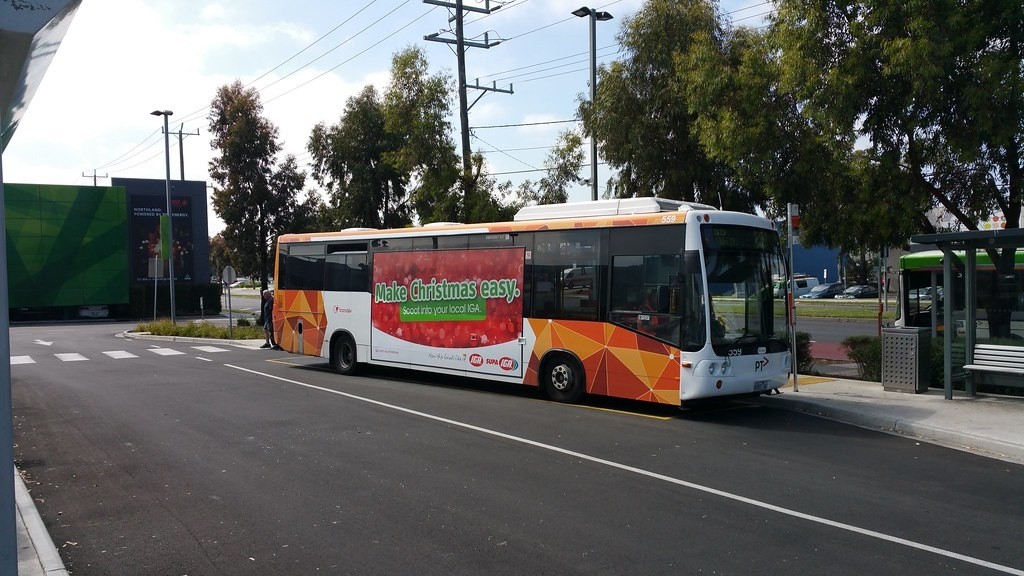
[570,6,615,199]
[150,110,176,323]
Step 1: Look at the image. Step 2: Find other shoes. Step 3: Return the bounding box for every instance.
[271,346,278,350]
[260,343,271,348]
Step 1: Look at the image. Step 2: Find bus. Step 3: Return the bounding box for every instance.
[893,247,1024,391]
[772,274,820,299]
[271,196,792,407]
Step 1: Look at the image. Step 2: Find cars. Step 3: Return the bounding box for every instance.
[834,284,879,299]
[799,282,848,299]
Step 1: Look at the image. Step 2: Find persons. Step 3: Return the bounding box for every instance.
[635,287,677,337]
[260,290,283,350]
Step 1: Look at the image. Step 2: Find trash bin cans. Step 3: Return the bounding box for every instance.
[880,327,932,395]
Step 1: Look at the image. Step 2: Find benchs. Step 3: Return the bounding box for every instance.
[962,344,1024,374]
